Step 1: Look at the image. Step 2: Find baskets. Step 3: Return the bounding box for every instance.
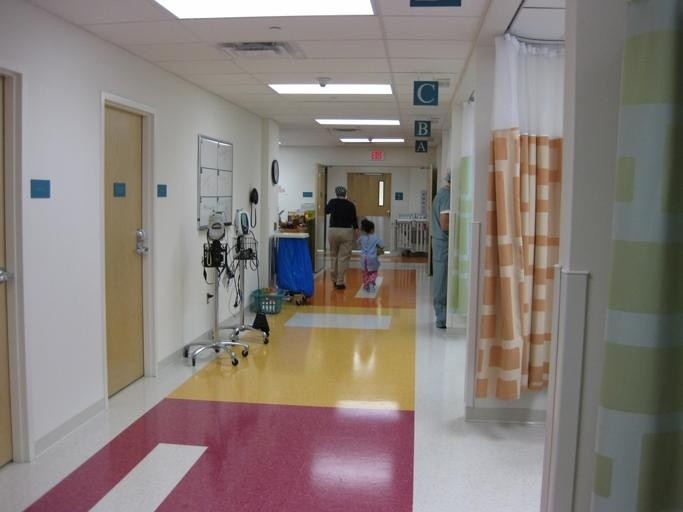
[251,288,287,314]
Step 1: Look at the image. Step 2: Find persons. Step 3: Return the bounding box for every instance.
[325,186,359,290]
[432,172,450,329]
[356,219,385,293]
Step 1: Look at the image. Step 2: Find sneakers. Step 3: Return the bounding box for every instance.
[335,285,344,289]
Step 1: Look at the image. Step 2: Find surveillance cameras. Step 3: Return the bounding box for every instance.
[318,80,328,88]
